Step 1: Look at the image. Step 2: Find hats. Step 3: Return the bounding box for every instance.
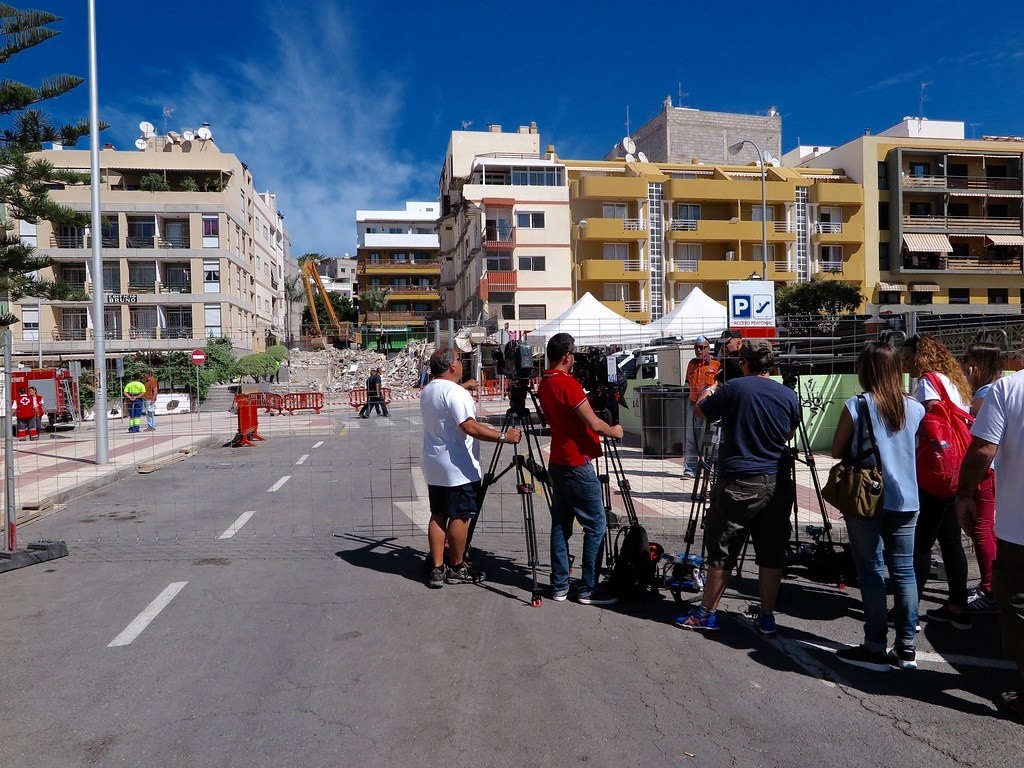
[718,329,742,342]
[737,340,773,364]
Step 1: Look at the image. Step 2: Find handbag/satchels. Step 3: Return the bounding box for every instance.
[820,393,883,518]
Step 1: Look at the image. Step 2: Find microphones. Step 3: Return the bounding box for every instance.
[604,347,623,357]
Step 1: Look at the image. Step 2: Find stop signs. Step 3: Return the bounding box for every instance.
[191,349,206,365]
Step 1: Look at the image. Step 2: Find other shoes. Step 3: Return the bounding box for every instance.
[384,413,391,417]
[552,592,568,601]
[577,591,618,604]
[681,474,690,478]
[359,413,368,418]
[129,429,138,432]
[144,428,155,431]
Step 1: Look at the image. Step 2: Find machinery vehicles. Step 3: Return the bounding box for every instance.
[301,260,358,350]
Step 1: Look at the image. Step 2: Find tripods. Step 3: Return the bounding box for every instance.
[677,420,744,588]
[463,408,574,606]
[592,414,660,598]
[740,414,841,575]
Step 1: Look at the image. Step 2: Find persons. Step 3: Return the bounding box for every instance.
[270,357,280,385]
[537,333,624,606]
[420,348,522,589]
[358,367,391,419]
[674,329,801,636]
[830,342,925,671]
[11,386,45,441]
[962,341,1003,614]
[124,373,146,433]
[143,370,157,431]
[954,369,1024,684]
[887,332,973,633]
[420,357,431,388]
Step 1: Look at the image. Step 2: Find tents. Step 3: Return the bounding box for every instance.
[523,285,728,370]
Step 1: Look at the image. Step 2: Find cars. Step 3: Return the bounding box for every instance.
[610,345,668,379]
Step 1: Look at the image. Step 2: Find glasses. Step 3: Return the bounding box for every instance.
[963,355,972,363]
[913,333,920,341]
[694,345,709,352]
[447,358,463,369]
[564,350,578,358]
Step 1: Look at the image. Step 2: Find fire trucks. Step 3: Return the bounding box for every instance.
[11,369,80,431]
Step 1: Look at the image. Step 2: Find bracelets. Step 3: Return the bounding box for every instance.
[957,493,974,501]
[706,387,714,394]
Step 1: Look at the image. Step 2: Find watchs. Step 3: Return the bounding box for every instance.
[498,432,505,443]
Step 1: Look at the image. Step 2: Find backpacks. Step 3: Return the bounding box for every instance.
[913,372,996,495]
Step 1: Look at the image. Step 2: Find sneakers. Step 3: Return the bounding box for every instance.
[756,614,776,634]
[886,608,922,631]
[446,563,487,585]
[431,563,449,587]
[676,607,720,630]
[835,644,890,673]
[968,583,988,596]
[927,607,973,629]
[888,641,916,668]
[968,598,1001,613]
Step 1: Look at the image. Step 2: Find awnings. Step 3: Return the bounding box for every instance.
[910,282,940,292]
[902,232,953,253]
[986,234,1024,245]
[875,281,908,292]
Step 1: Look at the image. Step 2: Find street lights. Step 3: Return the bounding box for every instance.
[728,140,769,281]
[574,220,587,301]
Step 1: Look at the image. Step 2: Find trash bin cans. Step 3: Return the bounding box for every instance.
[635,383,690,458]
